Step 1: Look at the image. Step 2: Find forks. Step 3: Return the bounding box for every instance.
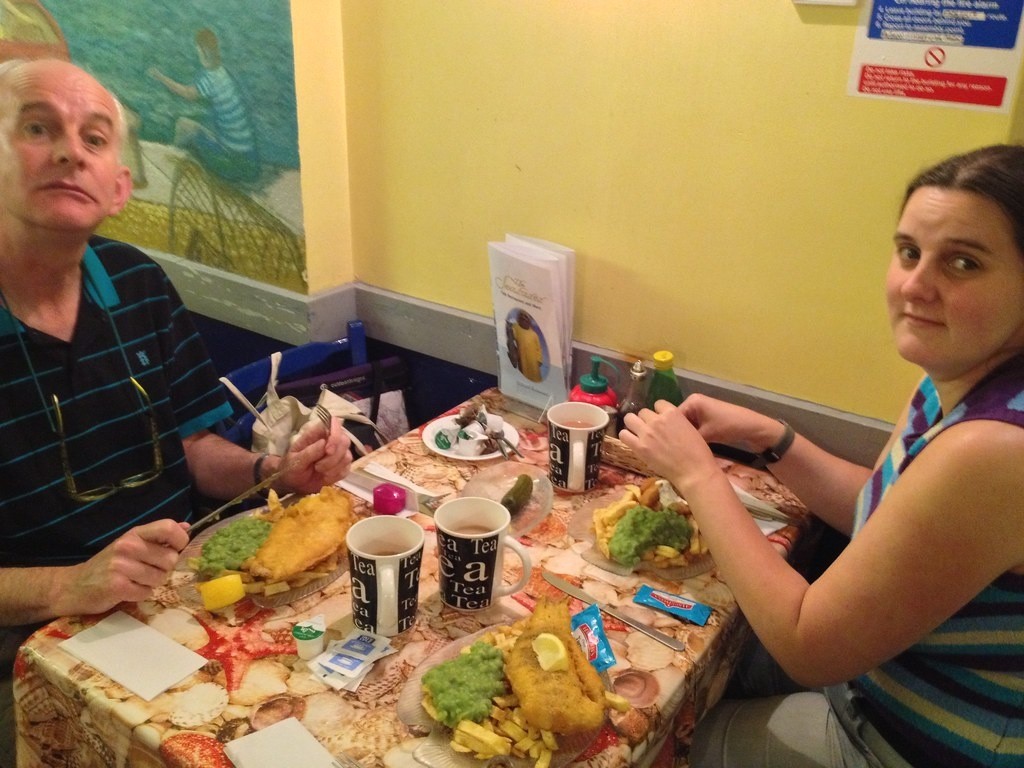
[330,750,363,768]
[354,468,451,502]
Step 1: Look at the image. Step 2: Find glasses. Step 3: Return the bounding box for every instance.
[49,376,165,504]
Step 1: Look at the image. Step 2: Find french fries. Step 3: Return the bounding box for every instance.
[186,489,339,596]
[593,484,708,568]
[421,615,630,768]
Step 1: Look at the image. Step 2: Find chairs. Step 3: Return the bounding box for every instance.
[217,319,368,510]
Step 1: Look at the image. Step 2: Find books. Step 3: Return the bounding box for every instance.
[487,234,576,410]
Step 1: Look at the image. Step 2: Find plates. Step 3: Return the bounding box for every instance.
[399,620,611,768]
[170,505,351,618]
[423,414,518,460]
[567,490,718,580]
[463,460,552,538]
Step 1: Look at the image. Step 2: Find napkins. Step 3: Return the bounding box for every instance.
[57,610,209,701]
[655,479,788,536]
[334,461,436,517]
[223,717,341,768]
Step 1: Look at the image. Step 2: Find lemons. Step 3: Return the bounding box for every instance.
[200,574,244,611]
[532,632,569,672]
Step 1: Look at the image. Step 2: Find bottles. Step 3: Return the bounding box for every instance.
[646,350,685,411]
[616,360,649,441]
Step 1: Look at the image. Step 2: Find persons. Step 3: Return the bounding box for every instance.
[0,58,352,629]
[511,308,544,382]
[617,143,1024,767]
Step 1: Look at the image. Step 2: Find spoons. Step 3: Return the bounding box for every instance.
[315,403,331,430]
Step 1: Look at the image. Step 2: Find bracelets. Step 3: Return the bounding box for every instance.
[254,452,273,501]
[757,419,794,466]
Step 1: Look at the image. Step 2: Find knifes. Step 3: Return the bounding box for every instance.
[541,570,686,651]
[165,460,301,549]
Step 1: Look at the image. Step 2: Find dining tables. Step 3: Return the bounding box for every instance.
[13,389,824,768]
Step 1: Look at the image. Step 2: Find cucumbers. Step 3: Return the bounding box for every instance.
[500,473,533,514]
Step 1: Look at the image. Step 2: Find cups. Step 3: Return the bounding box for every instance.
[434,498,530,612]
[347,516,426,636]
[547,402,611,494]
